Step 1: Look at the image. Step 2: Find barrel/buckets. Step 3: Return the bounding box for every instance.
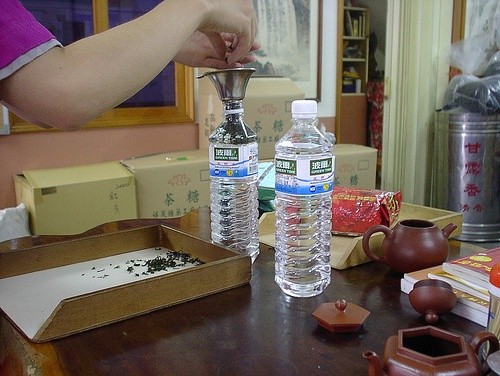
[431,110,500,243]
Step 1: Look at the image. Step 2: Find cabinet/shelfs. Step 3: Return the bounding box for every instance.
[339,5,371,146]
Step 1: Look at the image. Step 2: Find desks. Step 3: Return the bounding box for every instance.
[0,206,491,375]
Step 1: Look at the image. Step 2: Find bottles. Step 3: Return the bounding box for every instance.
[485,262,500,351]
[208,99,261,264]
[273,99,336,298]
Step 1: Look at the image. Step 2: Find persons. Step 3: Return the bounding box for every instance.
[0,0,260,133]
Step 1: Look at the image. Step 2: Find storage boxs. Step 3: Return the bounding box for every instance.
[197,76,306,161]
[117,149,212,219]
[13,160,138,236]
[332,144,379,190]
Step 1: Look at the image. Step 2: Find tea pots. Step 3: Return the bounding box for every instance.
[362,325,500,376]
[362,219,457,273]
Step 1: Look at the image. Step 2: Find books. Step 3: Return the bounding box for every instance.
[342,66,362,93]
[346,11,366,36]
